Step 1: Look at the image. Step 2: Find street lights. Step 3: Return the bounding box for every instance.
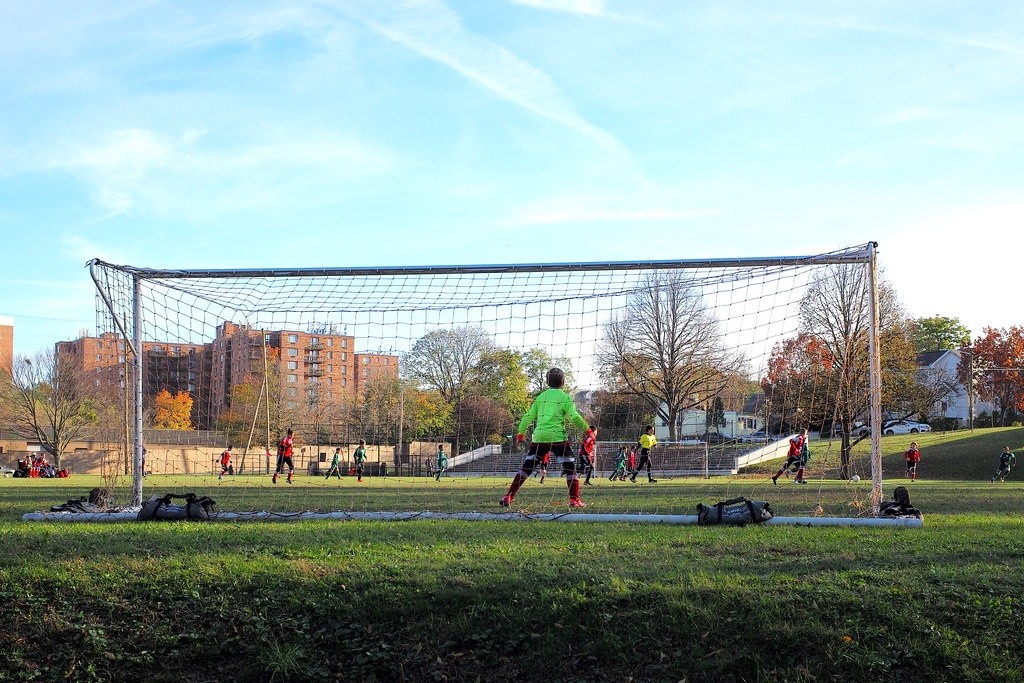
[960,352,974,429]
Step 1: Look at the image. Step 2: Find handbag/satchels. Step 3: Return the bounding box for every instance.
[138,492,216,522]
[696,497,773,526]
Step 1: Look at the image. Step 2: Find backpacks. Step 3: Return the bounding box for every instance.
[90,487,112,506]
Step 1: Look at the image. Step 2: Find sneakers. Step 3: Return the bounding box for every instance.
[571,495,586,508]
[501,495,512,508]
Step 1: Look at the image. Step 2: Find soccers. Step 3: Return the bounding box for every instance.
[853,475,860,482]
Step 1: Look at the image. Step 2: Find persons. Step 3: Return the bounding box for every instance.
[990,446,1016,484]
[608,445,636,481]
[771,428,807,485]
[904,442,920,482]
[425,456,433,477]
[433,444,448,481]
[785,439,812,484]
[326,448,342,479]
[216,445,233,479]
[272,428,295,484]
[561,426,597,485]
[499,368,595,507]
[142,445,147,477]
[534,452,550,484]
[354,439,367,482]
[21,452,55,478]
[630,424,658,483]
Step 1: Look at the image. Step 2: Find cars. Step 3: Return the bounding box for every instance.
[679,434,703,445]
[742,432,783,443]
[0,464,15,474]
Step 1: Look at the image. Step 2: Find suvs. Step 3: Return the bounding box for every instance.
[701,432,742,444]
[832,422,871,438]
[884,419,932,435]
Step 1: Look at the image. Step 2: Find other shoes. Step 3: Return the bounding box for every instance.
[584,480,593,485]
[288,479,294,485]
[608,476,657,482]
[795,477,807,483]
[358,479,364,482]
[785,471,789,478]
[772,476,777,485]
[273,477,276,483]
